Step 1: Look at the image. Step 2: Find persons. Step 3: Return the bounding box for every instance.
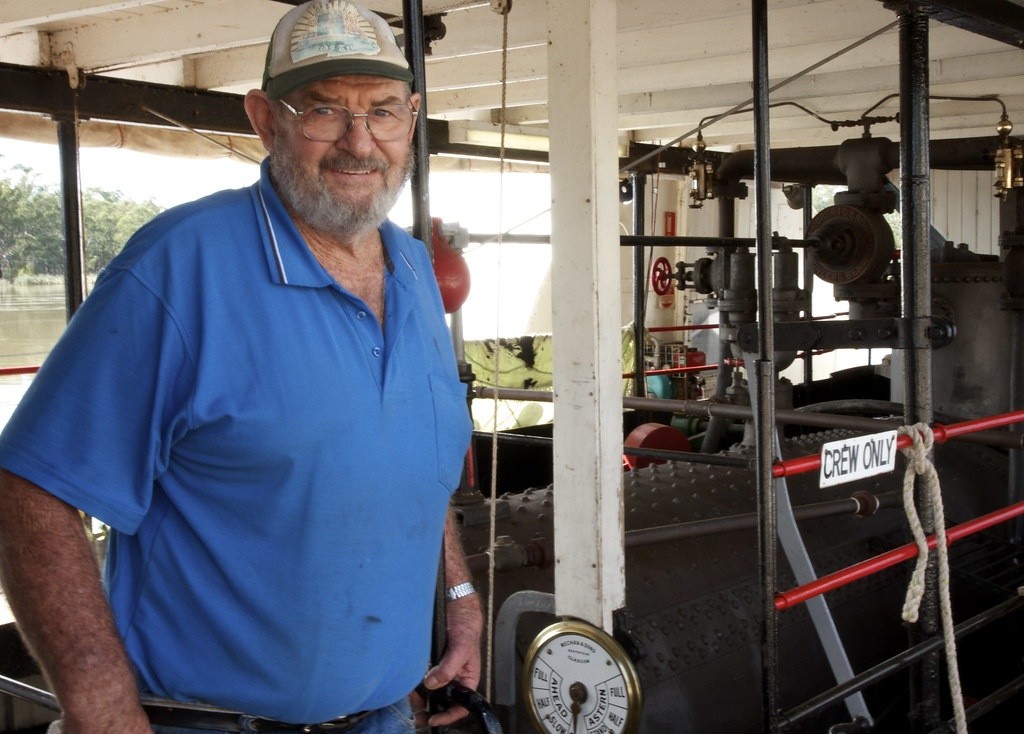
[0,0,486,733]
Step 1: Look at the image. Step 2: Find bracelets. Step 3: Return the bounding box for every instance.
[447,581,475,600]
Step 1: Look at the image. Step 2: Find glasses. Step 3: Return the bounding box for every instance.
[280,98,418,143]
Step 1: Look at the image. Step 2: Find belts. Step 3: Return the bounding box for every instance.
[142,704,370,734]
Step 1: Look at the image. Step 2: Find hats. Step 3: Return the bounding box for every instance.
[262,1,415,99]
